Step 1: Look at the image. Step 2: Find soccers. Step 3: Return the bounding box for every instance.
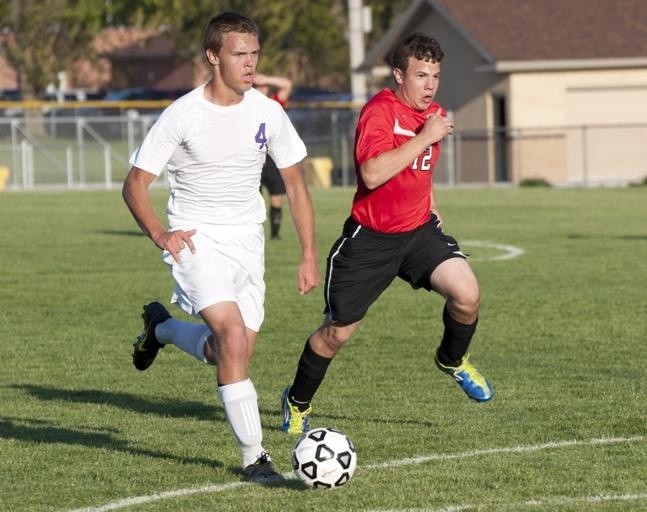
[292,428,357,490]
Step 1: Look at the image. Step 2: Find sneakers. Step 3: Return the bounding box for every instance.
[243,451,280,480]
[434,345,493,402]
[280,385,313,434]
[130,301,172,371]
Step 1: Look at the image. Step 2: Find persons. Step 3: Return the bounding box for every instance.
[121,13,321,481]
[252,72,294,240]
[283,33,494,434]
